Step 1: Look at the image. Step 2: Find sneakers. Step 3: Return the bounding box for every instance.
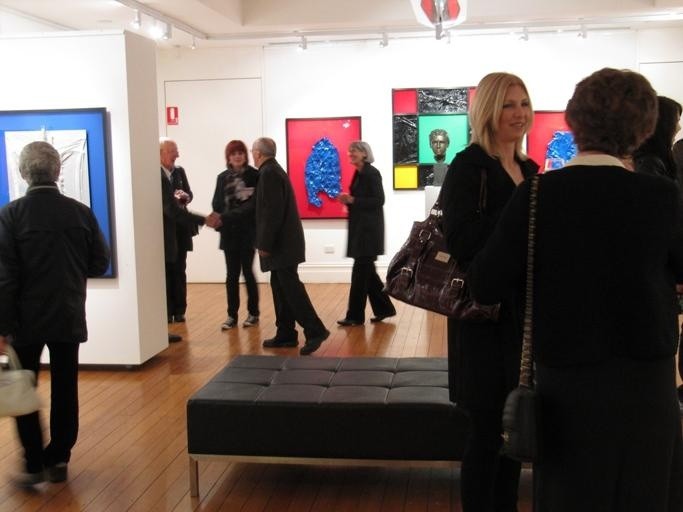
[370,307,400,323]
[242,313,261,326]
[167,314,185,323]
[221,315,238,331]
[13,467,44,489]
[47,463,69,484]
[337,316,368,327]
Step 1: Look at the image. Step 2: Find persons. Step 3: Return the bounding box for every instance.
[0,141,109,488]
[633,97,683,183]
[443,73,540,512]
[210,138,330,356]
[469,68,683,512]
[671,139,682,404]
[161,140,221,342]
[213,140,260,330]
[335,142,396,326]
[430,129,449,163]
[162,167,214,323]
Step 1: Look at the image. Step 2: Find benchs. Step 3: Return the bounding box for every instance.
[183,352,536,498]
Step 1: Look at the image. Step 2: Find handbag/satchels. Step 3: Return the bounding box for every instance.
[0,343,45,422]
[378,212,504,325]
[497,380,546,468]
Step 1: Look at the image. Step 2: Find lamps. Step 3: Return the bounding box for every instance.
[127,6,199,53]
[294,22,589,51]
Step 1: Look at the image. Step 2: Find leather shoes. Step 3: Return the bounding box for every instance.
[261,334,301,349]
[299,328,332,356]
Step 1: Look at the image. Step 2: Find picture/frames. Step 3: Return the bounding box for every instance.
[524,109,580,177]
[389,84,479,193]
[0,105,118,281]
[284,116,362,220]
